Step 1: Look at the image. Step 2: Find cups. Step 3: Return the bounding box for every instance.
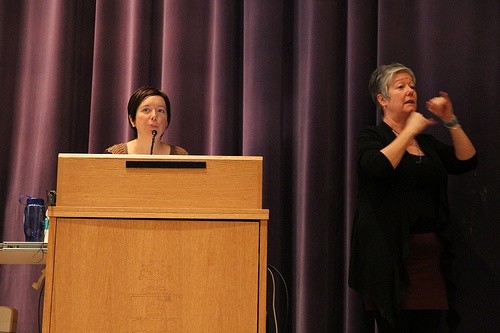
[19,194,45,242]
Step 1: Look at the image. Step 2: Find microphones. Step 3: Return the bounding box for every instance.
[151,130,157,154]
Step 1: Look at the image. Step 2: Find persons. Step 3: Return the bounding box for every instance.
[348,63,479,333]
[102,86,191,155]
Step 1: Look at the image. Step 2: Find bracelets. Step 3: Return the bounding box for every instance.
[444,120,460,129]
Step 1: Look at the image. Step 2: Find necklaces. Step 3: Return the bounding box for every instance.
[393,128,421,164]
